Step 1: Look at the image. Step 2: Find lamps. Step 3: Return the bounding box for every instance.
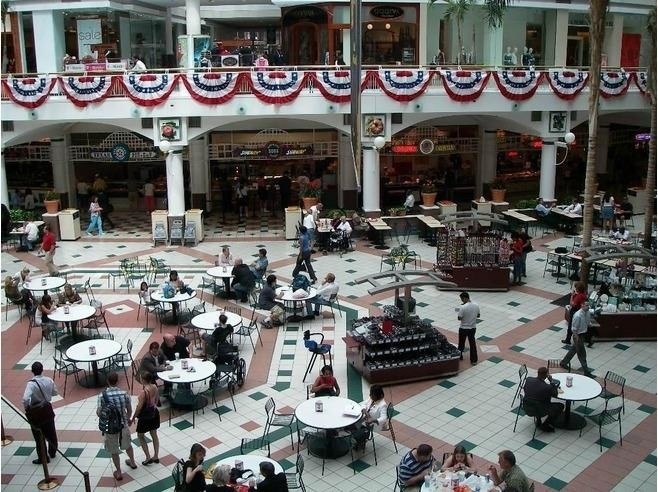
[372,137,385,152]
[160,141,173,157]
[554,132,576,148]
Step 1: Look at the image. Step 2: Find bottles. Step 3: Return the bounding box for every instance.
[425,469,462,492]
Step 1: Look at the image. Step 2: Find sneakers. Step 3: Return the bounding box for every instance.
[273,320,284,326]
[260,320,272,329]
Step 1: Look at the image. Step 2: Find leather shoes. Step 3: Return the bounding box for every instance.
[584,370,597,378]
[313,309,319,316]
[541,424,554,432]
[560,362,569,369]
[32,456,50,463]
[562,340,572,344]
[152,457,159,463]
[587,341,594,347]
[304,315,315,320]
[142,458,153,465]
[535,420,542,426]
[50,454,55,458]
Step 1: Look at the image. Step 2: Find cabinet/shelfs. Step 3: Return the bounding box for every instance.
[627,188,646,215]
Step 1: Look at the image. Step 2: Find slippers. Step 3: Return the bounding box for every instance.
[113,471,122,480]
[125,459,137,469]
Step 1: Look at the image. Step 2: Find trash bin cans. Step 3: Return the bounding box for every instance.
[184,208,205,242]
[151,209,169,241]
[436,202,457,231]
[471,199,492,227]
[285,205,302,240]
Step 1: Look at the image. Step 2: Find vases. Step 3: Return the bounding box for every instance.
[303,197,319,209]
[421,193,437,207]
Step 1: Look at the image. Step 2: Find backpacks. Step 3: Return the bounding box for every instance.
[291,274,311,293]
[99,390,124,434]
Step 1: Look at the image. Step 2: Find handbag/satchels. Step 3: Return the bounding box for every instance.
[315,388,335,396]
[25,401,54,425]
[136,401,154,420]
[164,285,174,298]
[180,282,186,294]
[352,417,370,443]
[230,468,253,484]
[293,263,306,276]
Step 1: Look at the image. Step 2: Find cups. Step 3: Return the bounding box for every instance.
[174,353,180,362]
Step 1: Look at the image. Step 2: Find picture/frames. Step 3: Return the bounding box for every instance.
[362,114,386,137]
[158,118,182,141]
[549,112,568,133]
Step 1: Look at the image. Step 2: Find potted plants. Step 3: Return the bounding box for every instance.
[44,191,61,213]
[491,179,508,203]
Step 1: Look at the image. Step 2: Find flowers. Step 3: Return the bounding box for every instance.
[421,179,437,193]
[298,178,321,198]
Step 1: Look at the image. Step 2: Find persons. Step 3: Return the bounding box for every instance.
[1,165,657,492]
[130,55,147,74]
[433,46,475,65]
[503,45,537,69]
[254,54,269,71]
